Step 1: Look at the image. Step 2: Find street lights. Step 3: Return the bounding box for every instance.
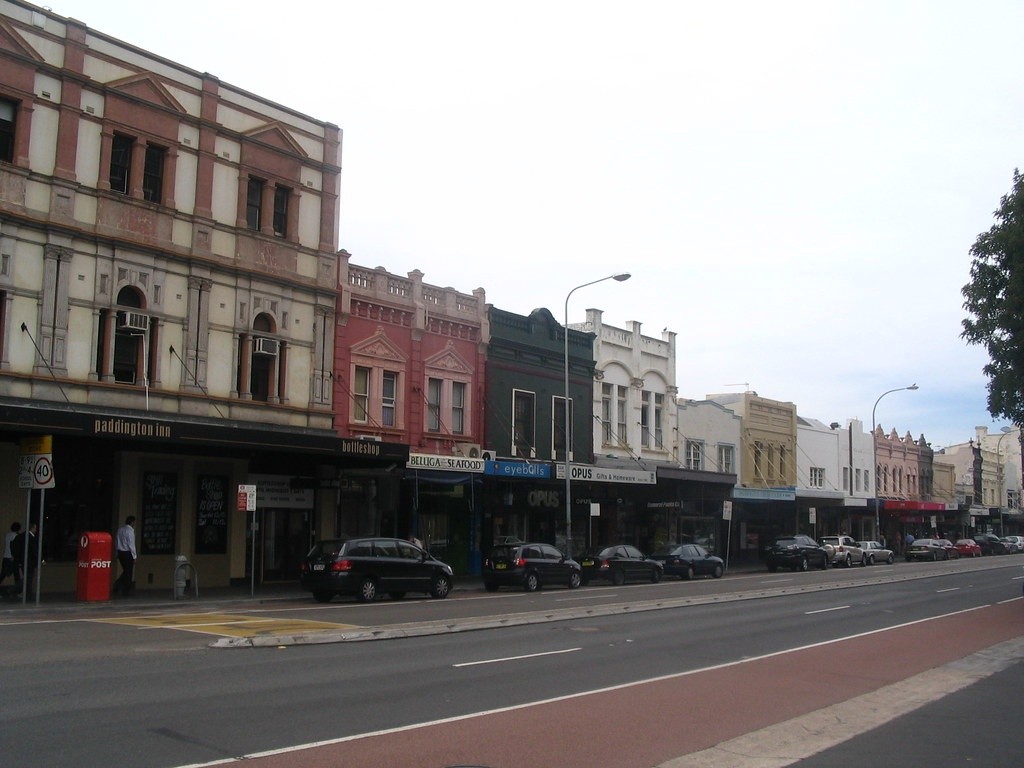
[997,428,1024,536]
[872,386,919,543]
[565,272,631,558]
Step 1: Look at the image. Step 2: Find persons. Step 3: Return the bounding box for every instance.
[992,527,1002,538]
[0,522,38,602]
[895,531,940,555]
[404,532,422,560]
[842,530,849,536]
[113,516,137,597]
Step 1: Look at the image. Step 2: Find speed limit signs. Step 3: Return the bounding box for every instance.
[33,458,52,485]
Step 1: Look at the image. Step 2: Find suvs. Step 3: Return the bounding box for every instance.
[815,534,867,567]
[763,534,828,572]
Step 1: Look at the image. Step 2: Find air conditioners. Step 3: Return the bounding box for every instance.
[355,435,382,442]
[252,338,279,356]
[456,443,480,458]
[481,450,497,461]
[119,312,149,331]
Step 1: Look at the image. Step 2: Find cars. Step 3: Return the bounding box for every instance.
[938,539,959,560]
[1000,538,1019,554]
[1005,535,1024,553]
[904,539,948,561]
[492,536,521,545]
[650,544,724,580]
[576,545,664,586]
[482,542,583,592]
[857,541,895,565]
[954,539,982,557]
[301,538,454,604]
[971,533,1005,555]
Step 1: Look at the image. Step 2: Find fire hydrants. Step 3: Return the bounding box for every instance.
[174,554,188,599]
